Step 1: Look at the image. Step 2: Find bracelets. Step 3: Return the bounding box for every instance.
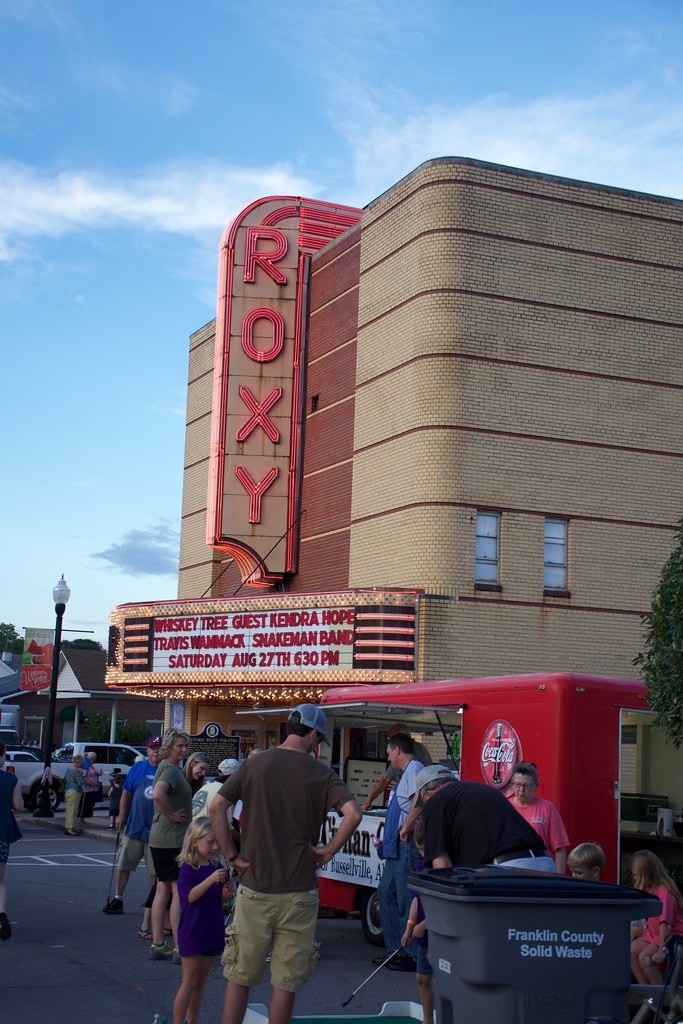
[407,919,415,925]
[225,852,241,863]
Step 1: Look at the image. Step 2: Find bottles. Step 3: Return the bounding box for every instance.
[447,733,454,759]
[674,807,683,838]
[455,734,461,760]
[451,734,459,760]
[494,723,502,780]
[151,1014,168,1024]
[370,834,382,850]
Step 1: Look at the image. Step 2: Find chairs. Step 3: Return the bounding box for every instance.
[584,934,683,1024]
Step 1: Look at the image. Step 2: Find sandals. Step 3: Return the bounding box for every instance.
[163,928,173,936]
[137,928,153,940]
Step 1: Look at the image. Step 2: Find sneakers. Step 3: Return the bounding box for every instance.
[171,948,181,965]
[149,940,175,959]
[102,898,124,914]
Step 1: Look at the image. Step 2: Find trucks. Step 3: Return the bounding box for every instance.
[0,704,21,747]
[313,673,682,942]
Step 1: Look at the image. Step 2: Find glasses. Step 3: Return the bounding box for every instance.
[511,776,537,790]
[150,744,162,750]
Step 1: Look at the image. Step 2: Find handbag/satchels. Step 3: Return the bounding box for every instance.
[95,782,104,802]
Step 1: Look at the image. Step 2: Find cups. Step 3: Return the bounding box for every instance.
[656,807,672,836]
[97,769,102,774]
[81,770,87,776]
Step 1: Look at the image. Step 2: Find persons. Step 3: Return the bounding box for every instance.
[207,704,363,1024]
[361,721,682,1023]
[0,744,24,941]
[101,726,263,1024]
[77,751,102,825]
[64,755,97,836]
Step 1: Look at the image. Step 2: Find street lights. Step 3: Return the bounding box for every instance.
[33,575,71,818]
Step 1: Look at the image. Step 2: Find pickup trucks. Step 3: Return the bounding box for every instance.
[0,743,149,815]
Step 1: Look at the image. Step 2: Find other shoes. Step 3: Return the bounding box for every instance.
[108,825,115,830]
[64,829,80,836]
[389,957,417,972]
[372,952,398,966]
[0,912,12,940]
[265,947,273,962]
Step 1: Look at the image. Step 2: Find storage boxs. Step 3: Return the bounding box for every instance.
[621,793,670,833]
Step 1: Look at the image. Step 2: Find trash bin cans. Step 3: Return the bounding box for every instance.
[405,865,662,1024]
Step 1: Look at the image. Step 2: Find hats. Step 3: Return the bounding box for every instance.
[288,703,331,747]
[412,765,456,808]
[147,736,162,748]
[218,758,240,775]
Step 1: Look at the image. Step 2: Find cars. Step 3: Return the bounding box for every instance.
[2,745,50,763]
